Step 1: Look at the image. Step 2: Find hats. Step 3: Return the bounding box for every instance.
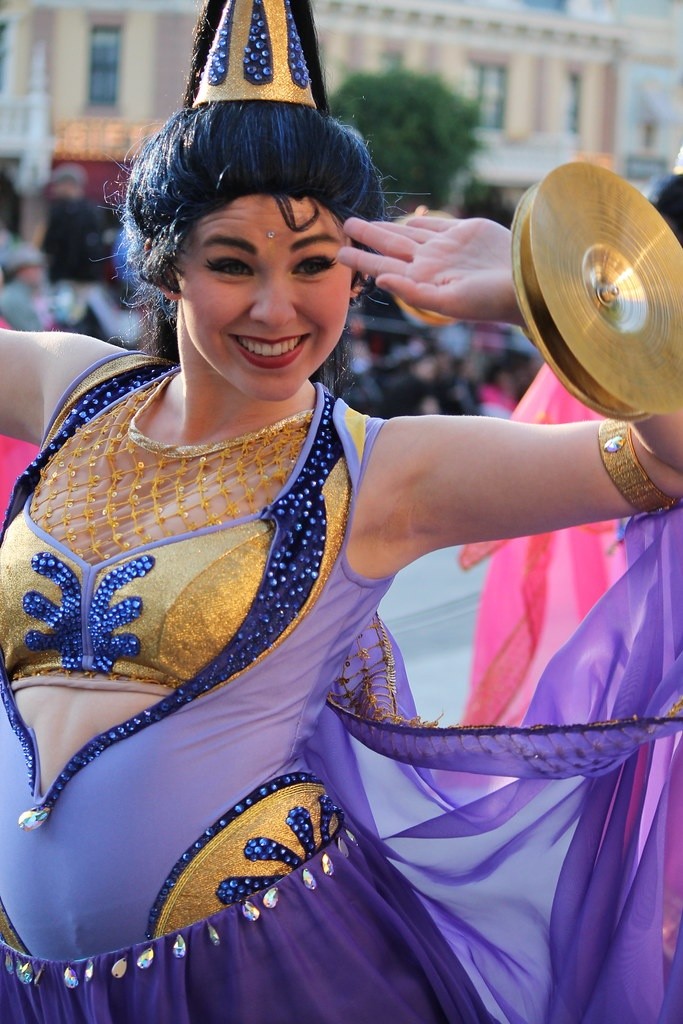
[49,164,87,187]
[5,244,47,274]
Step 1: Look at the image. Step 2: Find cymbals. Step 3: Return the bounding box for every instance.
[510,161,682,422]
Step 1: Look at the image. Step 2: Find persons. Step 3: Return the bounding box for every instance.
[0,165,545,430]
[435,145,683,793]
[0,0,683,1024]
